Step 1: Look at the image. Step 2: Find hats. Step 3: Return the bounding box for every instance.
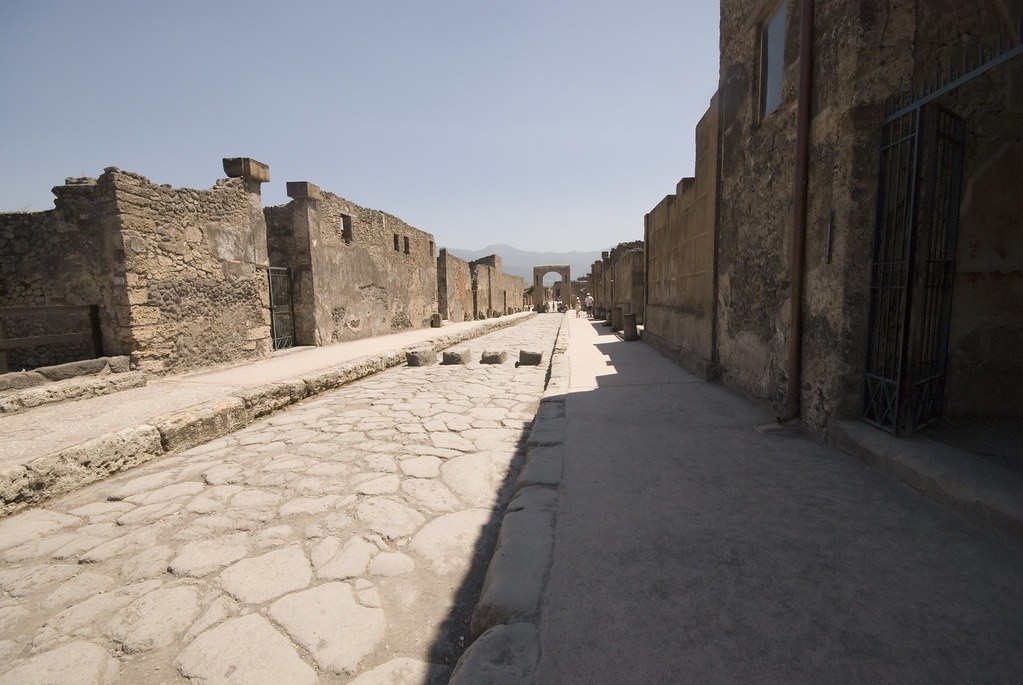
[587,293,590,296]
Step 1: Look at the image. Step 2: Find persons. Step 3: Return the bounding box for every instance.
[575,297,582,318]
[585,293,594,318]
